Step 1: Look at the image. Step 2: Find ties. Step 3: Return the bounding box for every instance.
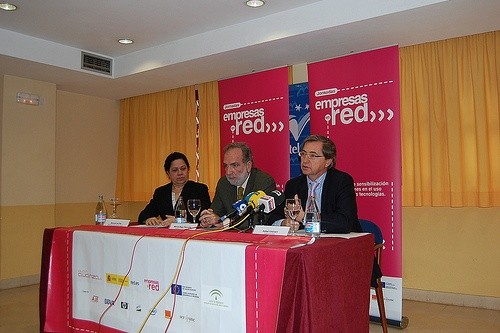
[237,187,244,200]
[308,181,320,223]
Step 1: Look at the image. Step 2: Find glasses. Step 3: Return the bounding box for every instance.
[298,152,326,159]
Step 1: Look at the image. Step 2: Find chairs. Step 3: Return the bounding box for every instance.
[358,219,388,333]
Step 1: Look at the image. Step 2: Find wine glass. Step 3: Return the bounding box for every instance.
[286,198,301,236]
[108,198,120,218]
[187,199,201,223]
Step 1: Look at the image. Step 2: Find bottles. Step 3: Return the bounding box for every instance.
[305,196,321,239]
[95,196,107,225]
[175,195,187,223]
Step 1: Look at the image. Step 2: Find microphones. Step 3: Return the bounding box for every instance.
[218,189,285,225]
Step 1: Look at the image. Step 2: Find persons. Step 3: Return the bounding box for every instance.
[266,134,382,288]
[199,142,285,230]
[138,152,212,226]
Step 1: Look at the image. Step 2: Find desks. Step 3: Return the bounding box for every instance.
[39,223,375,333]
